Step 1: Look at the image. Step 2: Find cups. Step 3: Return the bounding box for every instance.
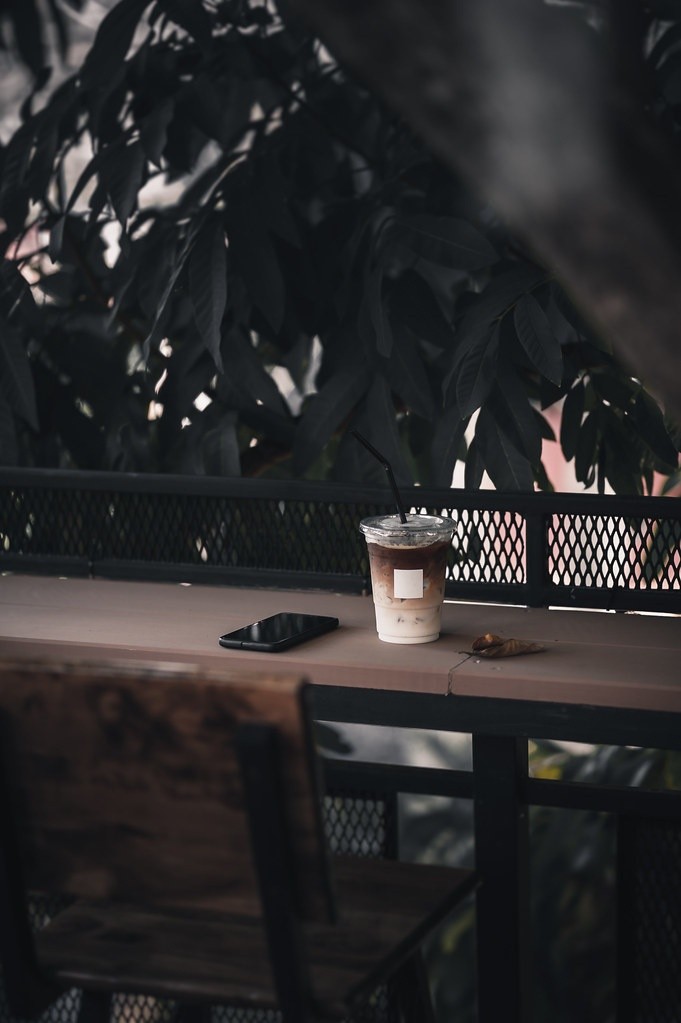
[358,512,456,644]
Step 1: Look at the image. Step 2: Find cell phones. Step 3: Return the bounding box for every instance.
[219,613,339,652]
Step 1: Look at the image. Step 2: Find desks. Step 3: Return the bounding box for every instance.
[0,570,681,1023]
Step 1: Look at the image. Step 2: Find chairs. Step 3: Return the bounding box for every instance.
[0,659,477,1023]
